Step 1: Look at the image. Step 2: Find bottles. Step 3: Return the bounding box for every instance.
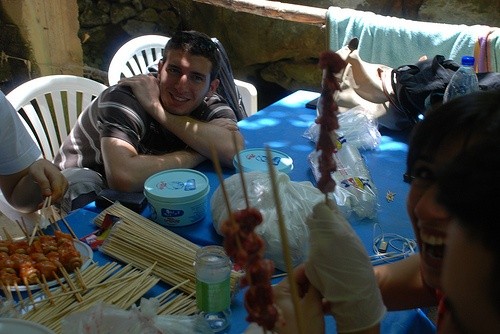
[442,56,479,105]
[194,246,234,332]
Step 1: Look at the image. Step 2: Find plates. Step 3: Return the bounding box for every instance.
[7,236,94,293]
[0,317,54,333]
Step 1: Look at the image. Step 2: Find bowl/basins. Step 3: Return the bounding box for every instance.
[233,148,294,175]
[143,168,210,227]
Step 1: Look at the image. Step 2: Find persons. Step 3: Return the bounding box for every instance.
[275,91,499,334]
[51,30,244,218]
[0,91,68,214]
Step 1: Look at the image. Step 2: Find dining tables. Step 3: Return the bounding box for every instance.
[0,89,442,334]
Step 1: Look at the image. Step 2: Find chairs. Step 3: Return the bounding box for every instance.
[0,75,109,231]
[107,35,257,121]
